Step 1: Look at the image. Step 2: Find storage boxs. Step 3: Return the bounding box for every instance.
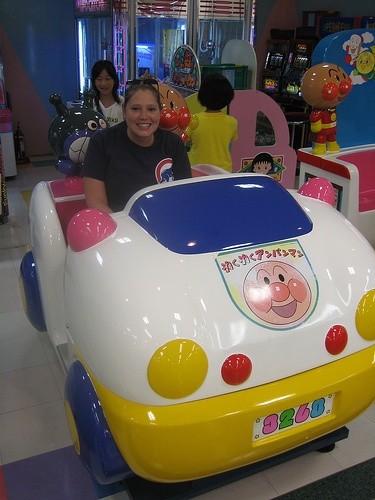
[201,63,249,91]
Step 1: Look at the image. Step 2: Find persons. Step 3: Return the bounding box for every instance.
[189,73,238,172]
[82,83,192,213]
[90,60,126,126]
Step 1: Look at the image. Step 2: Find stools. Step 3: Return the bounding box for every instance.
[283,113,309,148]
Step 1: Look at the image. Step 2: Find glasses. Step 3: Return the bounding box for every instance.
[125,79,159,101]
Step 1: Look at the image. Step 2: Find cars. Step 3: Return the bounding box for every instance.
[19,164,375,500]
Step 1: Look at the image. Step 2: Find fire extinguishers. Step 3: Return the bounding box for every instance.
[14,120,25,160]
